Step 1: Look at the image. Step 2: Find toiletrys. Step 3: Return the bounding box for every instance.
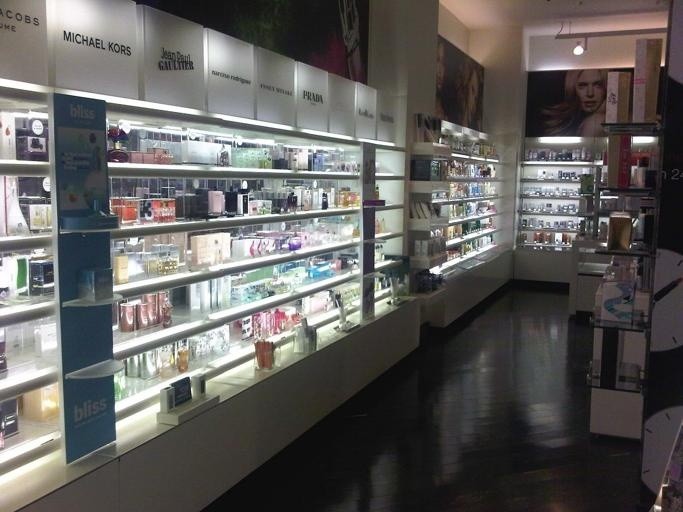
[638,204,655,241]
[314,152,324,172]
[415,237,443,258]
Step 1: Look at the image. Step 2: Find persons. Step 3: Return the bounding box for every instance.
[434,41,451,121]
[540,69,607,137]
[455,60,482,129]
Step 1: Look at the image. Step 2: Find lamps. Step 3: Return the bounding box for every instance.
[573,37,587,56]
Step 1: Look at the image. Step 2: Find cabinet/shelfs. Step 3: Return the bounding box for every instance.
[514,157,581,284]
[1,87,407,510]
[564,122,661,438]
[448,151,514,327]
[407,139,449,269]
